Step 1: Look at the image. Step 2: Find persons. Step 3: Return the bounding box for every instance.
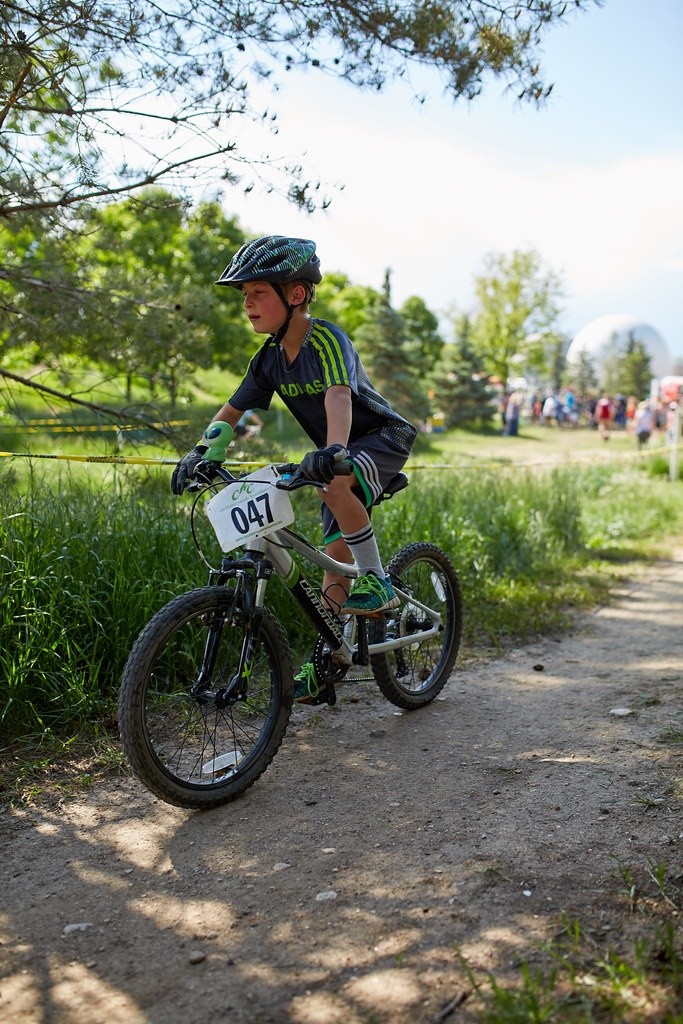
[530,386,683,429]
[667,401,679,444]
[593,397,612,440]
[635,401,653,449]
[171,235,418,707]
[500,389,525,437]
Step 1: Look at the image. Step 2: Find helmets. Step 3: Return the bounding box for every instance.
[214,235,322,291]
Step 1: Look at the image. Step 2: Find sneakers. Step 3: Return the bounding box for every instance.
[294,654,326,701]
[342,569,400,614]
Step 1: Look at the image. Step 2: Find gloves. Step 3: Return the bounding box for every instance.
[171,445,221,496]
[293,443,348,485]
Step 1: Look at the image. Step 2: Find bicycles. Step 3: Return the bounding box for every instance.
[117,420,465,810]
